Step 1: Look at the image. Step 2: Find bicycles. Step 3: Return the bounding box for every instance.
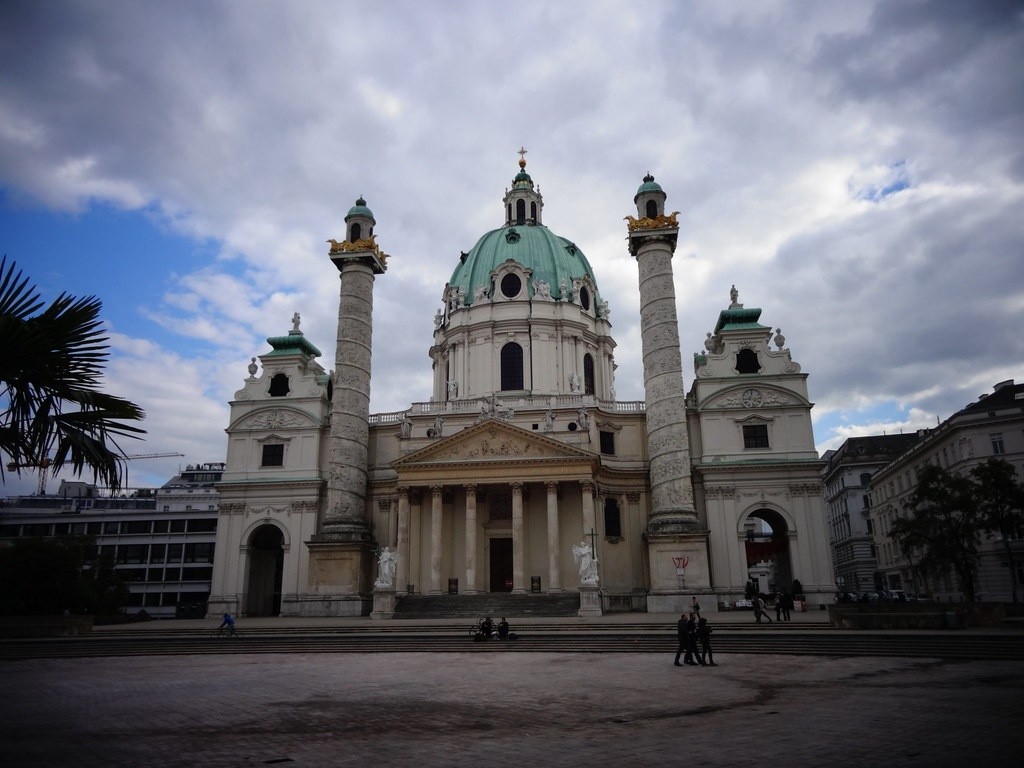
[469,618,498,637]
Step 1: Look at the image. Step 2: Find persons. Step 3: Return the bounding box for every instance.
[572,541,600,587]
[745,582,794,622]
[480,617,510,639]
[374,546,400,587]
[395,279,618,439]
[833,590,911,605]
[217,612,236,637]
[674,596,721,667]
